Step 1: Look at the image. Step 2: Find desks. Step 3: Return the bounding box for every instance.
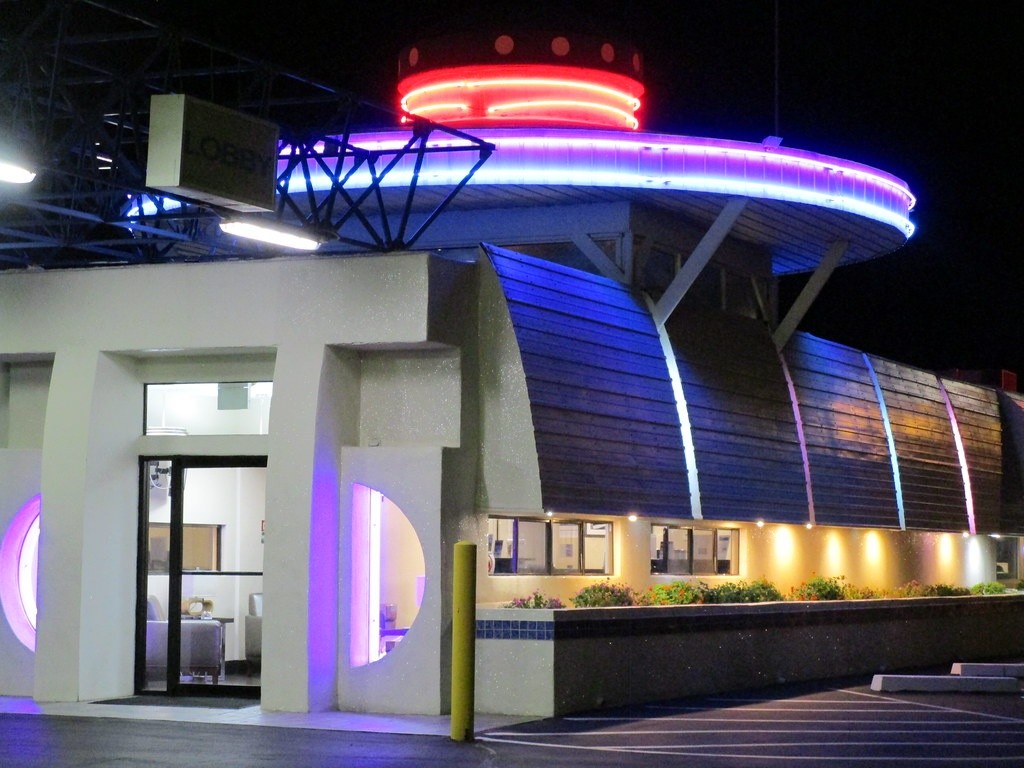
[380,628,410,657]
[180,617,235,685]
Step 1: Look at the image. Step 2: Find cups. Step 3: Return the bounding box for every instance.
[495,540,503,557]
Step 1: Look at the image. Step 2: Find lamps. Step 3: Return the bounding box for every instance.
[220,215,321,252]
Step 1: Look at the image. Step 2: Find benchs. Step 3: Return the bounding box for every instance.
[147,596,223,685]
[245,593,263,677]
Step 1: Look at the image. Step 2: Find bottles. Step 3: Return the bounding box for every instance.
[488,534,495,574]
